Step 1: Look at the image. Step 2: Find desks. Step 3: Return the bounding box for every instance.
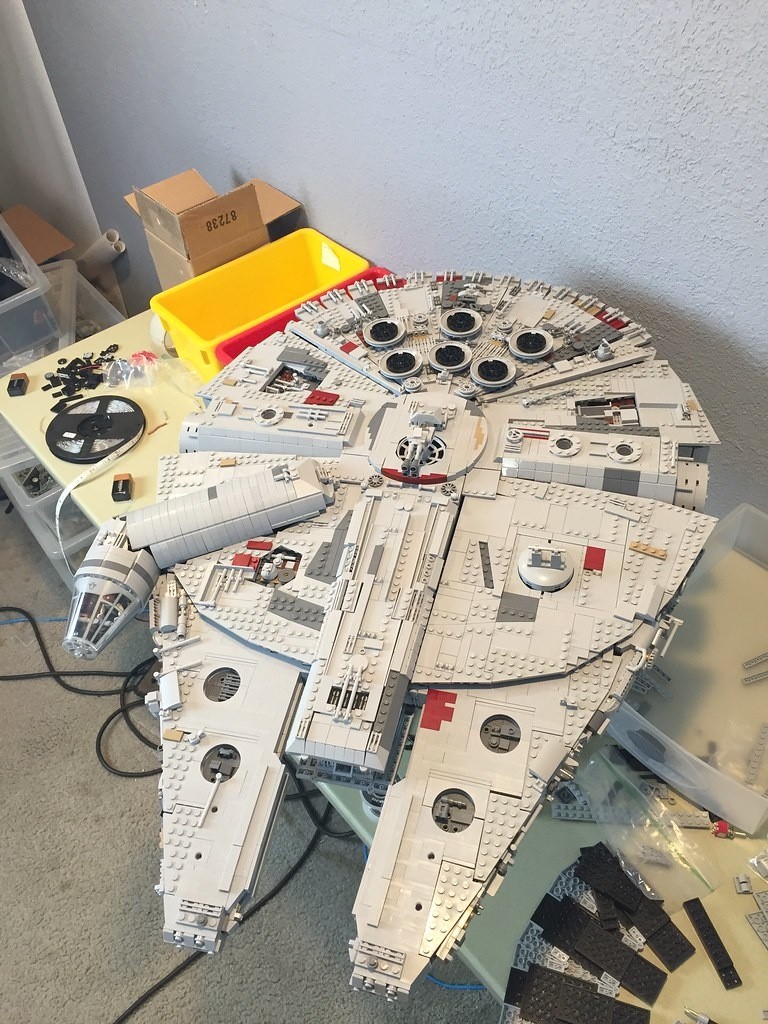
[1,310,768,1024]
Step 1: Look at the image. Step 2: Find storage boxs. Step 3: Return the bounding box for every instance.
[0,168,407,592]
